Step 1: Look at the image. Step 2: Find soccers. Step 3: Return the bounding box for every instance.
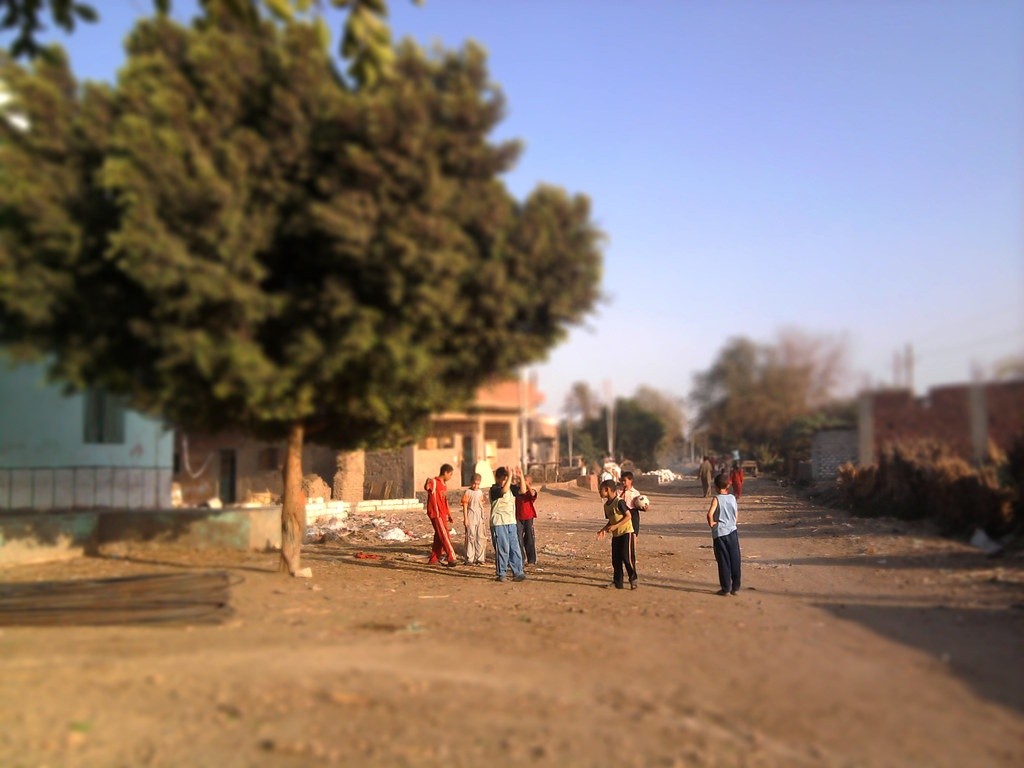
[635,494,650,509]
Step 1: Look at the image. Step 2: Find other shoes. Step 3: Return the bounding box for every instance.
[730,590,740,595]
[603,582,617,589]
[717,590,730,596]
[464,562,482,566]
[495,575,506,581]
[428,561,444,566]
[526,563,535,567]
[513,574,526,582]
[631,584,637,589]
[448,560,465,567]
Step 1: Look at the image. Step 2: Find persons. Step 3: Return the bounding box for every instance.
[461,473,486,566]
[697,452,723,498]
[721,461,743,500]
[489,466,537,582]
[621,472,649,572]
[424,464,465,567]
[707,474,741,596]
[596,479,638,590]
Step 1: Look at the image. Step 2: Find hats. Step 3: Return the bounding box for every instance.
[496,466,508,477]
